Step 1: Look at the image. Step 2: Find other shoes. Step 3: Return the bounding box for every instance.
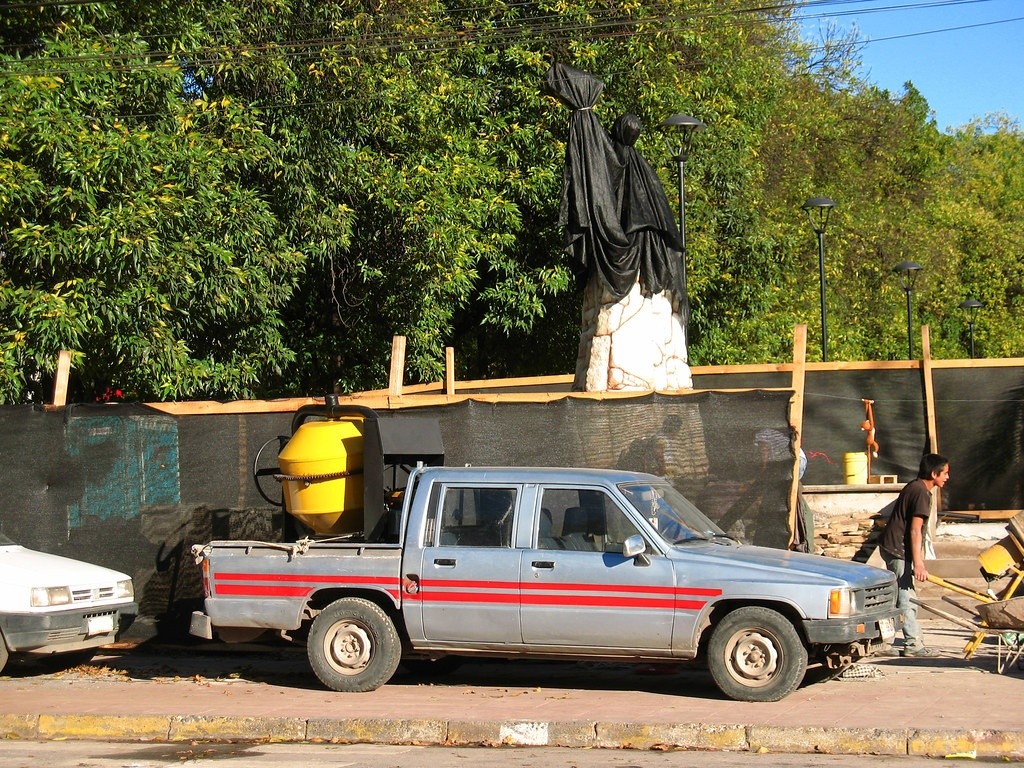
[874,645,900,657]
[904,642,941,657]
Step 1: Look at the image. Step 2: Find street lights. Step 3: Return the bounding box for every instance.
[798,197,837,362]
[892,261,924,359]
[654,114,707,366]
[956,299,986,359]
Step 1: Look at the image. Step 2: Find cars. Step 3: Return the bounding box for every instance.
[0,532,139,678]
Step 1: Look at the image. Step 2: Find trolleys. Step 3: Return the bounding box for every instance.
[909,594,1024,675]
[909,534,1024,661]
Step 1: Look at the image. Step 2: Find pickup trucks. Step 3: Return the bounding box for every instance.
[190,461,908,702]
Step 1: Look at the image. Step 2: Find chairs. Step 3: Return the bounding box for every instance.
[537,508,559,550]
[560,508,598,552]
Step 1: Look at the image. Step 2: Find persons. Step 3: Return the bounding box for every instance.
[749,427,808,481]
[878,454,950,658]
[637,414,682,487]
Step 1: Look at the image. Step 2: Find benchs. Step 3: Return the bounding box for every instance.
[440,522,511,546]
